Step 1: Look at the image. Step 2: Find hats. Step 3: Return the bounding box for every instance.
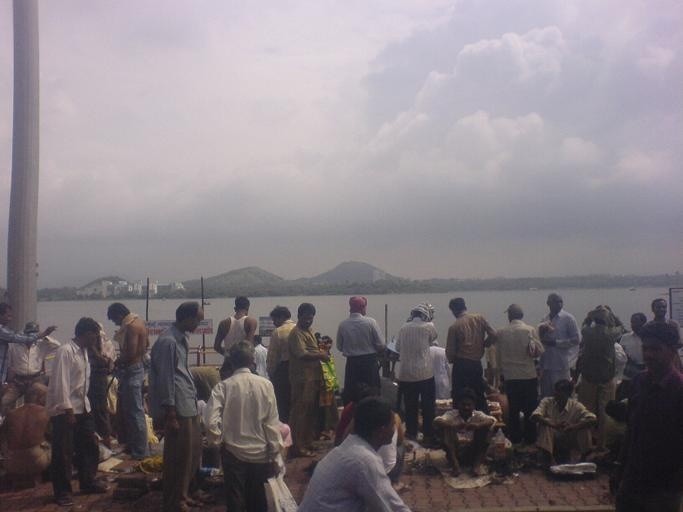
[349,296,367,307]
[24,324,39,334]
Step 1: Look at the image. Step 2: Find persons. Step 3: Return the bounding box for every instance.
[0,294,339,461]
[43,314,112,507]
[292,394,414,511]
[201,337,284,512]
[146,298,206,512]
[602,318,683,511]
[570,299,683,452]
[336,294,597,483]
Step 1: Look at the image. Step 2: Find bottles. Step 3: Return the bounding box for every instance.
[493,427,505,463]
